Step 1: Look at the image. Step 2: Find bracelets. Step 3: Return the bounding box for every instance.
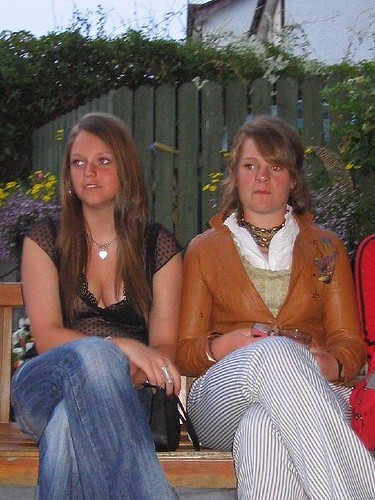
[105,336,112,340]
[335,358,344,382]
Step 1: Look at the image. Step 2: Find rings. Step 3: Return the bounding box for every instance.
[162,366,174,384]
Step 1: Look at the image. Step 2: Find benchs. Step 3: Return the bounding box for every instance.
[0,280,239,490]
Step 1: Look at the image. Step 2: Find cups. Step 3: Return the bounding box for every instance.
[278,327,313,349]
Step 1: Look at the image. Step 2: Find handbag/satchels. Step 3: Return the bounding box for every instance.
[350,375,375,452]
[142,383,201,453]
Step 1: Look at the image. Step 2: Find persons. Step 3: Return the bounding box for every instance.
[176,114,375,500]
[356,232,375,368]
[6,115,184,500]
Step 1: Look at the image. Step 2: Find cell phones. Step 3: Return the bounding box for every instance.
[251,323,271,338]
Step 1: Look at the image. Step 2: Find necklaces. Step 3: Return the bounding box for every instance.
[238,211,290,249]
[89,236,116,260]
